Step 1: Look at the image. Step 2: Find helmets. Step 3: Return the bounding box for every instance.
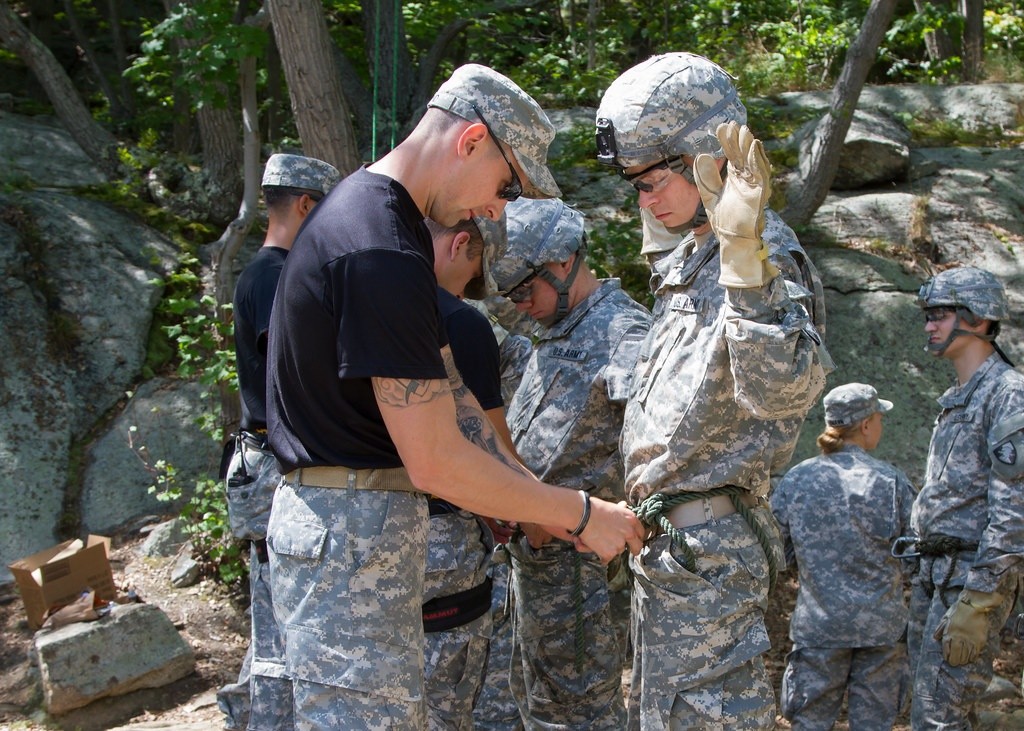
[920,268,1009,320]
[490,192,585,297]
[595,51,747,168]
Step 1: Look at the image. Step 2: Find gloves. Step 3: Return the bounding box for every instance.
[640,208,683,257]
[933,589,1008,666]
[694,120,781,288]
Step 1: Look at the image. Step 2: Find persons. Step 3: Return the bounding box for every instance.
[891,267,1024,731]
[594,52,836,731]
[216,154,340,731]
[769,382,919,731]
[266,63,644,731]
[492,198,653,731]
[421,215,534,731]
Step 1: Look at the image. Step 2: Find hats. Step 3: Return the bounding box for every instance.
[822,382,895,426]
[473,211,509,296]
[427,62,562,198]
[260,154,341,196]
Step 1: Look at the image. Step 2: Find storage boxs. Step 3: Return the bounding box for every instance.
[8,533,117,630]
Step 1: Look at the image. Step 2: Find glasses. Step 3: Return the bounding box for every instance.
[924,307,954,322]
[629,169,674,193]
[472,105,524,202]
[508,269,539,304]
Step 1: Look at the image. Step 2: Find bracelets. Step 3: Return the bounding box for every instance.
[567,491,591,536]
